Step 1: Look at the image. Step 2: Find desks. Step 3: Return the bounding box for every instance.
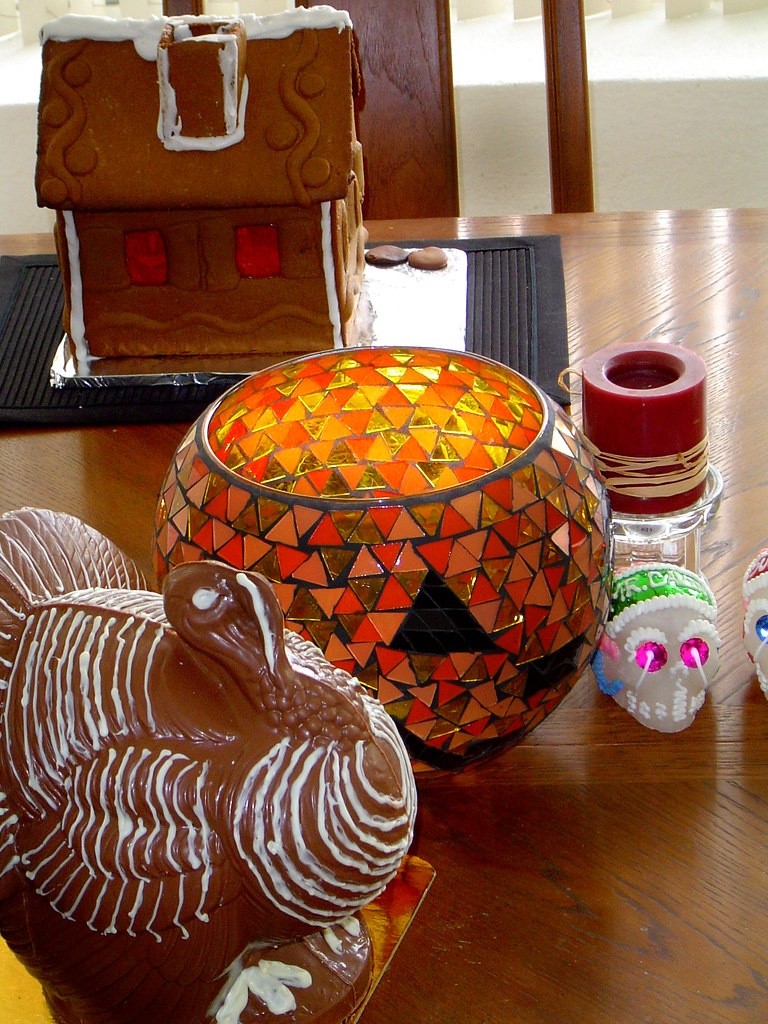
[1,204,768,1024]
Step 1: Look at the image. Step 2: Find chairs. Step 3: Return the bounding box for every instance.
[160,0,595,223]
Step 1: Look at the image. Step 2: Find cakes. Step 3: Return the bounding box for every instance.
[33,6,369,378]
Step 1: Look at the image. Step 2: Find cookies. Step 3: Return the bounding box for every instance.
[408,247,448,270]
[365,245,410,264]
